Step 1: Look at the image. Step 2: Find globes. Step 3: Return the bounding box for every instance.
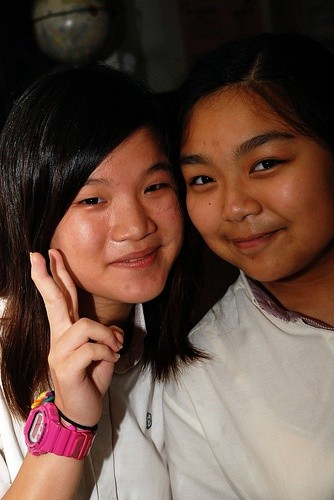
[31,1,119,68]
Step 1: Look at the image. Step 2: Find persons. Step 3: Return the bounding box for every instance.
[142,33,334,500]
[1,66,186,500]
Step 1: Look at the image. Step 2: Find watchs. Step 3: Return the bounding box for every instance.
[24,403,97,462]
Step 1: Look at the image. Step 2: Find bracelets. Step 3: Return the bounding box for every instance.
[48,390,99,432]
